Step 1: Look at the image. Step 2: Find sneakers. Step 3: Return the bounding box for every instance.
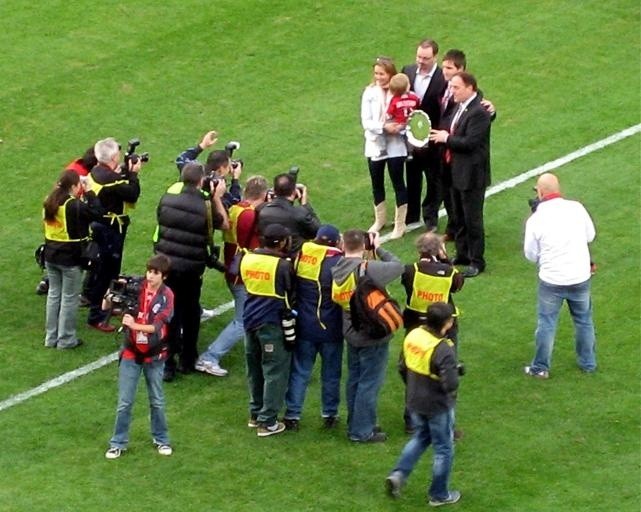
[77,292,122,346]
[385,476,399,498]
[403,425,415,433]
[369,425,386,443]
[324,415,337,426]
[162,359,228,381]
[153,438,173,456]
[36,282,48,294]
[453,429,462,439]
[248,418,297,437]
[525,366,549,378]
[428,491,461,506]
[106,447,127,459]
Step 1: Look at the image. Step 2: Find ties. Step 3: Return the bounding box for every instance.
[446,104,462,163]
[440,87,451,112]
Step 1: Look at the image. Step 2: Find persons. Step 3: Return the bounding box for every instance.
[42,137,141,349]
[522,172,601,381]
[99,255,178,463]
[240,174,402,445]
[383,300,460,506]
[402,233,462,436]
[153,131,269,379]
[359,38,498,284]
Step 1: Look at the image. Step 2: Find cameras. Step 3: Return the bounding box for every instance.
[124,139,149,163]
[203,170,226,192]
[81,177,89,185]
[289,165,304,198]
[364,230,377,251]
[264,188,275,201]
[277,309,298,350]
[226,140,244,171]
[528,187,540,212]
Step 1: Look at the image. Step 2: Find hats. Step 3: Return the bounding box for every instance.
[264,224,288,241]
[318,225,339,241]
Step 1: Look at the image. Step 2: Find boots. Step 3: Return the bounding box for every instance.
[391,204,407,239]
[369,201,386,232]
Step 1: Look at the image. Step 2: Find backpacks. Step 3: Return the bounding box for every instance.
[350,264,405,340]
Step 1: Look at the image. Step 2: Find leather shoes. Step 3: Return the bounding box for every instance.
[450,255,482,278]
[425,225,450,242]
